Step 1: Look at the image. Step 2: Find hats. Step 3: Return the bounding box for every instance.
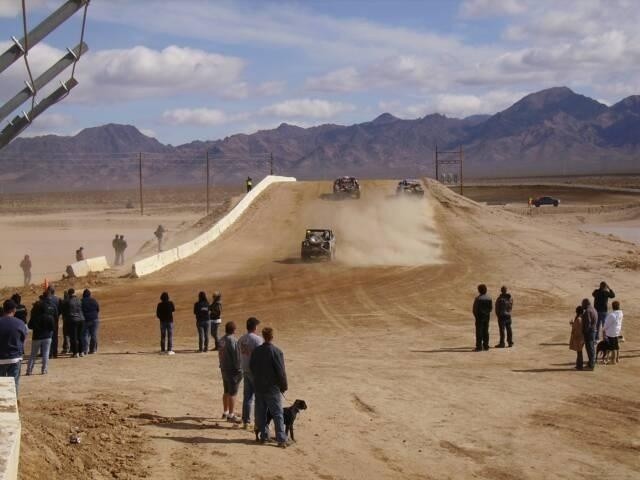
[246,317,261,326]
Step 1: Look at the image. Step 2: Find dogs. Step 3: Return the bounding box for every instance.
[595,335,625,363]
[253,398,308,444]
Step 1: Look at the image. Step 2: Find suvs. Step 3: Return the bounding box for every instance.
[300,228,337,261]
[333,174,361,200]
[397,176,424,198]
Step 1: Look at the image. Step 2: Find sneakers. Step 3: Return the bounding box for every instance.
[39,349,97,359]
[25,371,48,376]
[575,339,620,372]
[160,348,218,356]
[222,414,296,449]
[472,343,513,352]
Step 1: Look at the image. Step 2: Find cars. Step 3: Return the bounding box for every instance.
[529,195,561,207]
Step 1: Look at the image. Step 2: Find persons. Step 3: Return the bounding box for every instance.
[156,291,176,356]
[0,284,101,400]
[75,246,87,261]
[19,254,33,287]
[194,291,211,354]
[249,326,298,449]
[471,284,494,353]
[112,234,119,253]
[246,176,252,192]
[236,316,264,430]
[115,235,129,265]
[154,224,166,252]
[567,306,586,371]
[602,300,624,364]
[495,285,515,349]
[207,292,223,351]
[581,298,600,372]
[218,321,242,422]
[591,281,616,342]
